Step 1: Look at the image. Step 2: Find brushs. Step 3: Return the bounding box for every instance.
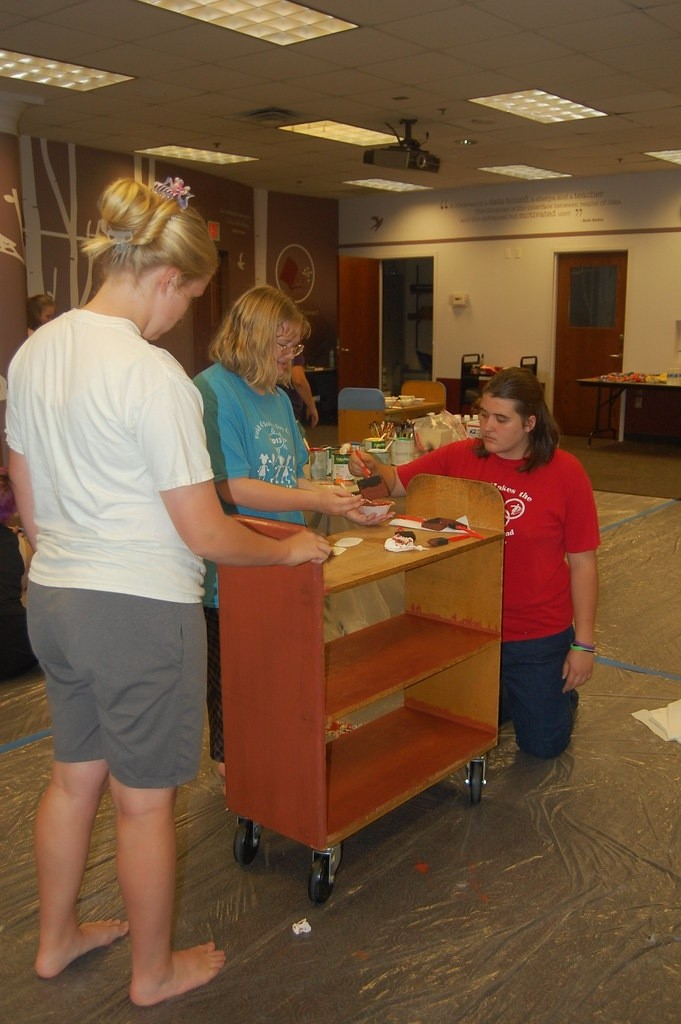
[395,514,456,531]
[355,449,389,501]
[448,520,482,539]
[427,535,470,547]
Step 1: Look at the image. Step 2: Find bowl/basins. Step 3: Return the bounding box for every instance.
[360,502,391,517]
[385,395,426,407]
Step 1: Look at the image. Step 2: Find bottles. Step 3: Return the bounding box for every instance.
[309,437,415,482]
[454,413,479,431]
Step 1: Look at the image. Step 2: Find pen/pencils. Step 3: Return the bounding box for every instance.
[368,419,415,437]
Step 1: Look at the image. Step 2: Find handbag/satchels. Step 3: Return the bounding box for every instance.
[410,408,467,450]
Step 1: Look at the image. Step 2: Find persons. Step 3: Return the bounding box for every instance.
[4,177,333,1008]
[278,340,318,427]
[190,284,395,778]
[26,293,55,338]
[347,367,601,760]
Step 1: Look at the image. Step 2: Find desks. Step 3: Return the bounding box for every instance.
[577,377,681,444]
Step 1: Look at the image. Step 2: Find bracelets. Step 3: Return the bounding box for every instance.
[570,640,595,652]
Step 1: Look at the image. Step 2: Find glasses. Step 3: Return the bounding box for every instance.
[277,342,305,356]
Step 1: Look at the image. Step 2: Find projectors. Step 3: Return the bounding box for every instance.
[363,147,440,174]
